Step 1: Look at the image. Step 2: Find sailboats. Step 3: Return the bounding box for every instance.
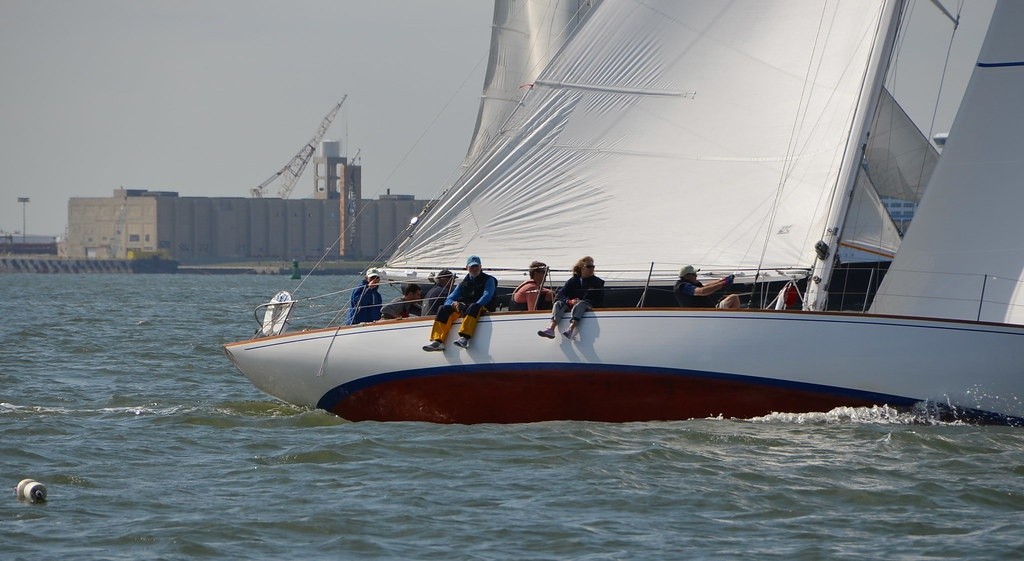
[223,0,1024,426]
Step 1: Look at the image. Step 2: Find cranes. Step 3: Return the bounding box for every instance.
[252,92,350,198]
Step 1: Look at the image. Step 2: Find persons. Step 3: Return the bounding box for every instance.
[674,265,740,309]
[538,256,606,338]
[508,261,556,312]
[422,256,498,352]
[380,283,422,320]
[346,267,383,325]
[420,270,458,315]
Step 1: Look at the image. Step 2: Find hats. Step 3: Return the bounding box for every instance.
[366,268,380,278]
[467,255,480,266]
[438,271,458,278]
[678,265,700,277]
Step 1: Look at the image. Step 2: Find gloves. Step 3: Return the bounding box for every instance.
[722,274,734,288]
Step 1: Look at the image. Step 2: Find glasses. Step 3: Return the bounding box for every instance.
[583,264,595,269]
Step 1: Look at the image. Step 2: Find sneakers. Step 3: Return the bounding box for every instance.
[538,328,555,339]
[453,336,470,349]
[422,340,445,351]
[563,327,573,339]
